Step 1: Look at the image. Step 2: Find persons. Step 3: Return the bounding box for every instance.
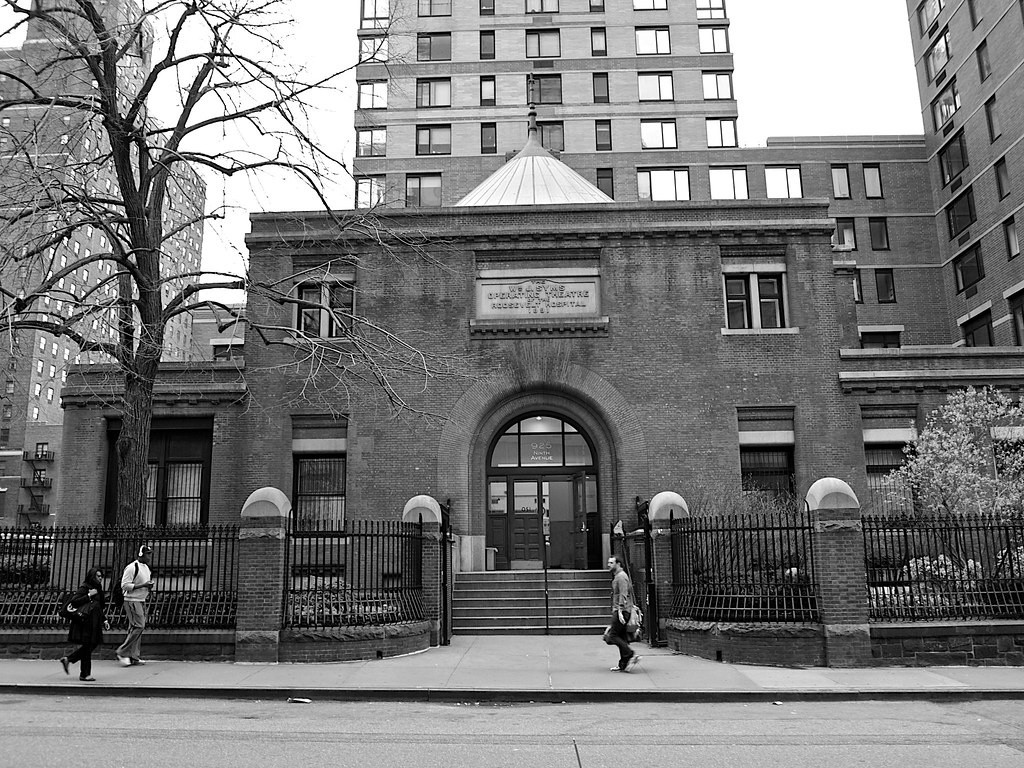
[60,567,110,681]
[608,556,642,673]
[115,546,154,666]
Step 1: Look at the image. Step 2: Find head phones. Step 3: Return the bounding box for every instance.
[138,545,144,556]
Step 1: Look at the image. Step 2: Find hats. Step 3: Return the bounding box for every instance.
[138,545,153,554]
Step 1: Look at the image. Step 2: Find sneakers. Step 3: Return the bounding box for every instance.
[626,656,641,672]
[79,675,95,681]
[60,656,69,675]
[611,665,622,672]
[115,652,146,665]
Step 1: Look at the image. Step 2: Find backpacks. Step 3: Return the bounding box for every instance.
[112,576,124,606]
[627,605,643,632]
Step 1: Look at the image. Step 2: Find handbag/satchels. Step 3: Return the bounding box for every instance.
[60,592,97,623]
[602,625,644,645]
